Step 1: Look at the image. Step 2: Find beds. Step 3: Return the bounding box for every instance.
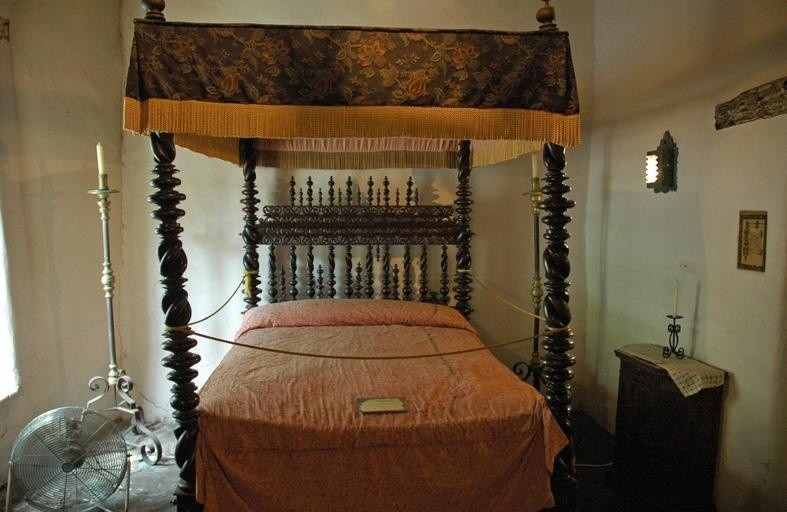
[196,325,551,509]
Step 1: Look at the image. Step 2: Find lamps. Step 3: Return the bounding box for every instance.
[643,150,674,188]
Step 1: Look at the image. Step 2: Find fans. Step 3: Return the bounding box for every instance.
[5,405,131,510]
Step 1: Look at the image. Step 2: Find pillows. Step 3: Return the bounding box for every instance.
[235,298,471,330]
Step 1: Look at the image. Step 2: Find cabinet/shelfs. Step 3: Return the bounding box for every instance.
[612,342,729,511]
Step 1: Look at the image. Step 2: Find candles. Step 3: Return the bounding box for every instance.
[671,278,679,317]
[94,140,106,176]
